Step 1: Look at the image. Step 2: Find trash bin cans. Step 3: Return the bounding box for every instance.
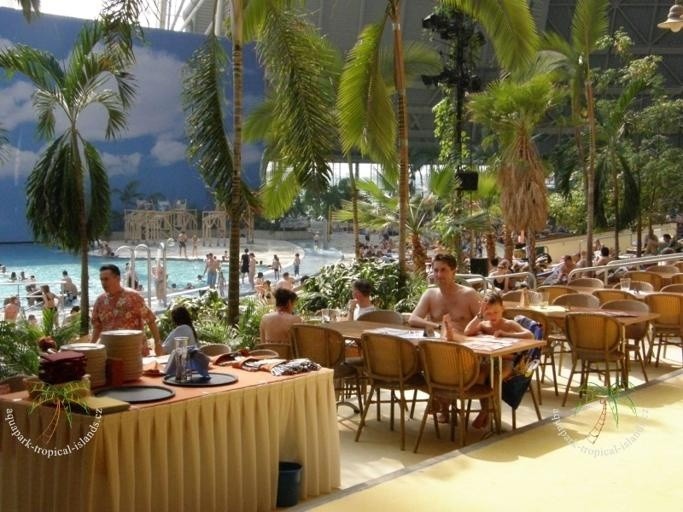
[276,461,303,507]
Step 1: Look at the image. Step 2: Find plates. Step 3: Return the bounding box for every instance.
[61,343,105,390]
[100,329,143,382]
[162,371,238,386]
[92,385,175,404]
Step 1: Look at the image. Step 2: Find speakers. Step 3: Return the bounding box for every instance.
[454,171,479,191]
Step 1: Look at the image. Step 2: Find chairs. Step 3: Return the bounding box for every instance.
[413,338,502,456]
[254,344,291,362]
[659,284,683,292]
[199,343,231,362]
[287,322,366,425]
[591,289,639,307]
[623,271,663,292]
[552,293,599,375]
[671,273,683,284]
[501,290,543,304]
[599,298,650,391]
[356,310,403,325]
[536,286,578,305]
[562,312,628,407]
[644,293,683,368]
[566,278,604,288]
[502,309,546,430]
[353,332,441,451]
[646,265,679,273]
[612,281,653,292]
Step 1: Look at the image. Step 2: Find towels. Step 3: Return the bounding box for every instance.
[164,344,212,381]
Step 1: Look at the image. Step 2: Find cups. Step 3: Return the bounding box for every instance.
[175,337,187,383]
[620,278,631,293]
[538,292,550,310]
[186,355,192,383]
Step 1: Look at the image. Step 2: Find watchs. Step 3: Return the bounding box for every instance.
[476,312,483,321]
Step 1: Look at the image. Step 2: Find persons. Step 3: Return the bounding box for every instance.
[40,285,62,331]
[26,285,44,307]
[96,240,118,259]
[151,259,168,308]
[346,279,376,322]
[162,306,198,353]
[489,259,512,290]
[293,253,300,278]
[0,263,37,284]
[123,262,145,291]
[594,247,616,269]
[4,296,21,324]
[276,272,293,289]
[643,227,683,254]
[58,270,79,306]
[253,272,276,306]
[556,255,578,282]
[259,288,304,345]
[408,253,483,423]
[239,248,251,285]
[197,274,203,281]
[190,233,201,257]
[202,253,227,299]
[272,253,280,281]
[247,253,256,292]
[178,230,188,257]
[357,233,396,261]
[464,292,533,429]
[90,265,163,356]
